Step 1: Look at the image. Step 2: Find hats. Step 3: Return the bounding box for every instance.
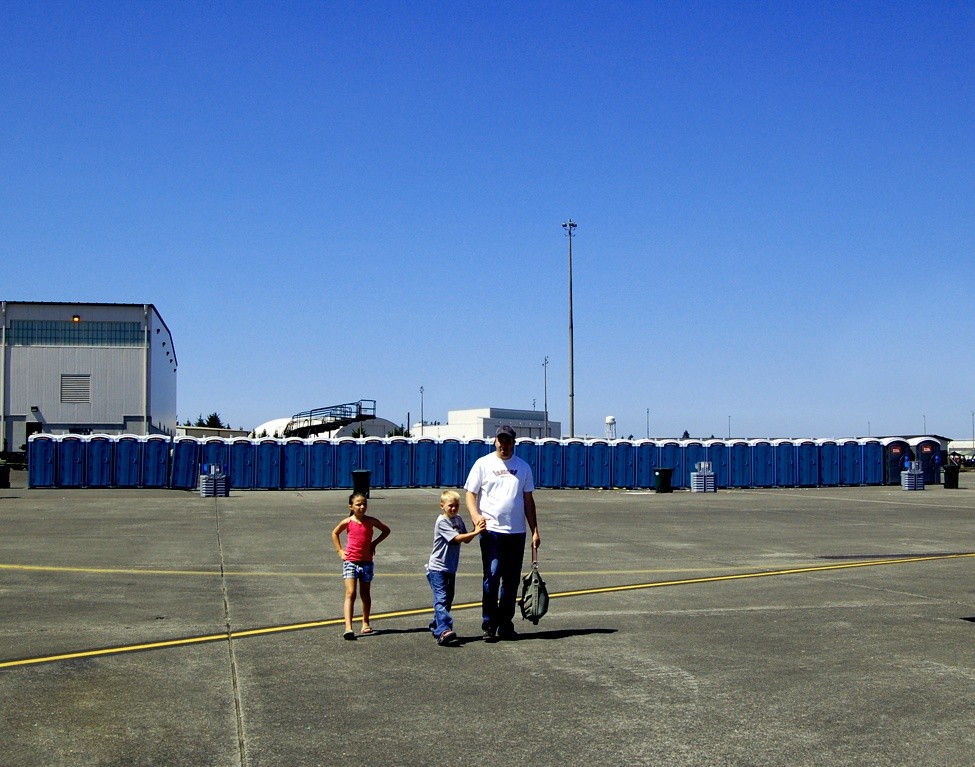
[495,425,516,438]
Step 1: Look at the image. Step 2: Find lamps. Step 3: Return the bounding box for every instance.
[73,315,80,322]
[31,407,38,412]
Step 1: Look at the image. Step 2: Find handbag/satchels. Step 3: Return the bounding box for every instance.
[518,543,549,626]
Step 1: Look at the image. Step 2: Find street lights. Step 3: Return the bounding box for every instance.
[541,355,549,437]
[561,218,578,439]
[419,385,424,437]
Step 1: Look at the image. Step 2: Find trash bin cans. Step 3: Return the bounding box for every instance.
[943,465,960,489]
[654,468,674,493]
[351,470,372,498]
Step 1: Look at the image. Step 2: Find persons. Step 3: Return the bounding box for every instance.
[464,425,541,640]
[427,490,485,646]
[332,493,391,640]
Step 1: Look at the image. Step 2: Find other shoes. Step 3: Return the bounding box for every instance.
[360,627,373,634]
[438,630,456,646]
[343,631,354,640]
